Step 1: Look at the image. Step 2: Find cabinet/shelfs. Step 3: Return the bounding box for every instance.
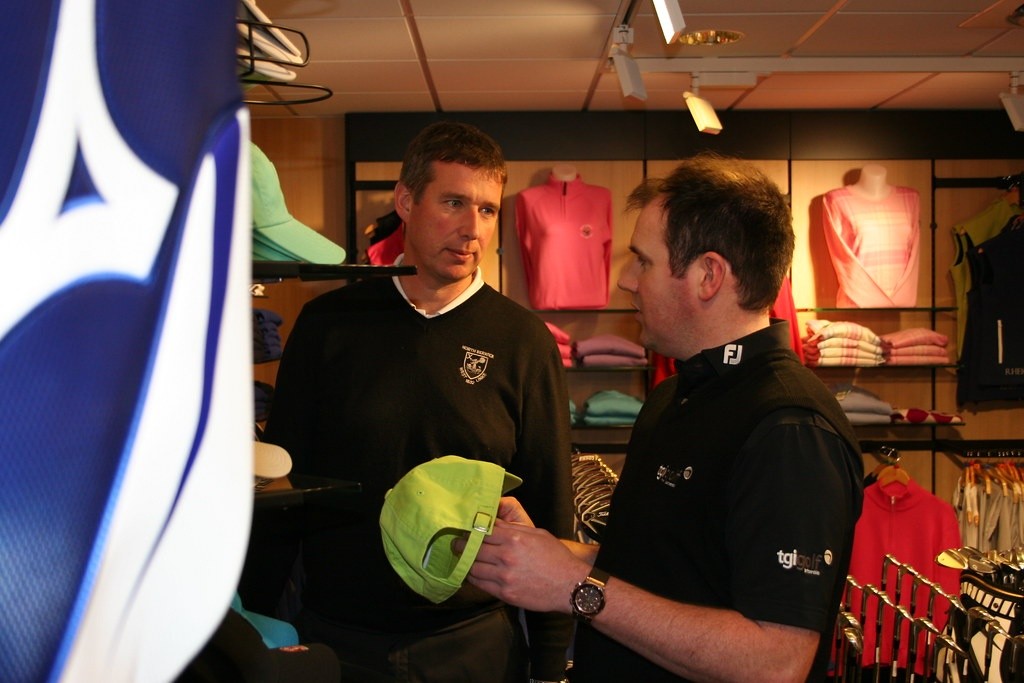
[344,107,1023,453]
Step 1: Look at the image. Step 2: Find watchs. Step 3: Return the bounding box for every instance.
[571,567,611,623]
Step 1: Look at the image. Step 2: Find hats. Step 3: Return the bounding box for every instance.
[375,454,522,607]
[248,138,348,270]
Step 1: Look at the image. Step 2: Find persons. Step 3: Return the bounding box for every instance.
[514,163,614,311]
[822,165,921,307]
[238,120,574,683]
[454,150,864,683]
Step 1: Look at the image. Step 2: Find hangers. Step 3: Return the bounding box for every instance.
[570,455,621,527]
[961,451,1024,525]
[871,449,911,490]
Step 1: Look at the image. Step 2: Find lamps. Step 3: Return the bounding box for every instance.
[682,71,722,134]
[653,0,685,45]
[609,44,647,101]
